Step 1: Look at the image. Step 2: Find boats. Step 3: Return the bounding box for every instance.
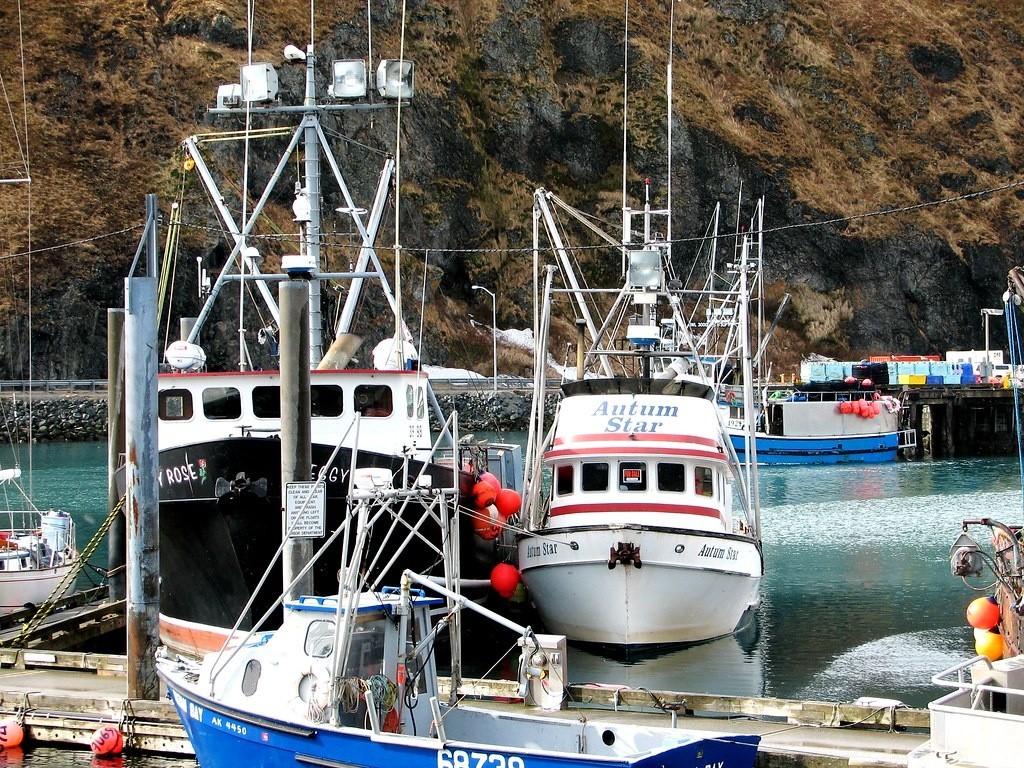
[0,467,80,617]
[137,0,1024,768]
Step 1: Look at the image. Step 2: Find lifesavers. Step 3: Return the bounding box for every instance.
[726,392,737,403]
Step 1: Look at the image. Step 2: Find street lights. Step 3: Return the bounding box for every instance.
[472,286,498,392]
